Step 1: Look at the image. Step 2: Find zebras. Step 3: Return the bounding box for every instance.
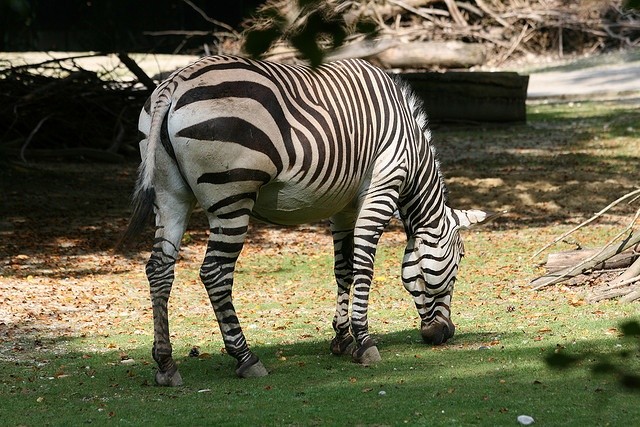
[111,55,487,386]
